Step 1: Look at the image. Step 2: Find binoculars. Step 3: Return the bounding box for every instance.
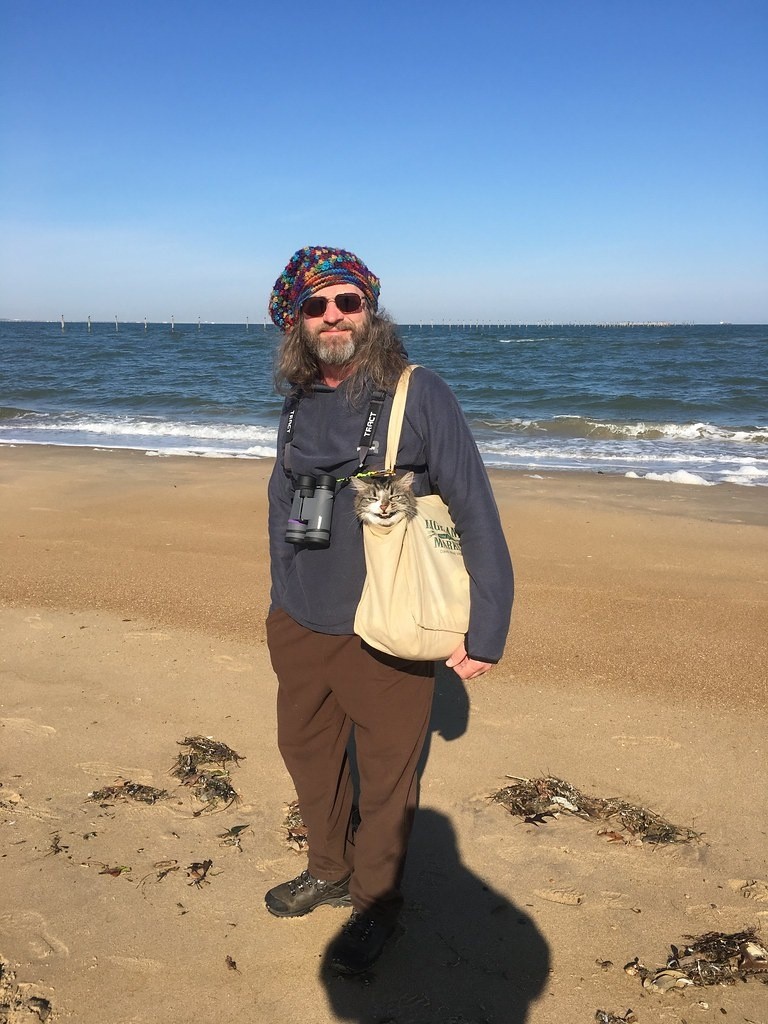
[284,475,335,548]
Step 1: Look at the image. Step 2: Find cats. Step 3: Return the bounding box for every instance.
[346,472,420,532]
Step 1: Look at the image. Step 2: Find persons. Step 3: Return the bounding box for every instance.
[265,245,514,978]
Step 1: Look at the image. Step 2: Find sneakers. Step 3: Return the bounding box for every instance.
[264,868,353,918]
[328,907,398,979]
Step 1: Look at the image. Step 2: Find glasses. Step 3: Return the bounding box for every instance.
[300,292,366,317]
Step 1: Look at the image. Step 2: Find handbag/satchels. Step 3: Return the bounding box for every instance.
[353,365,472,662]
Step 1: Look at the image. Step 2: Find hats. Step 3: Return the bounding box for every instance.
[268,245,381,337]
